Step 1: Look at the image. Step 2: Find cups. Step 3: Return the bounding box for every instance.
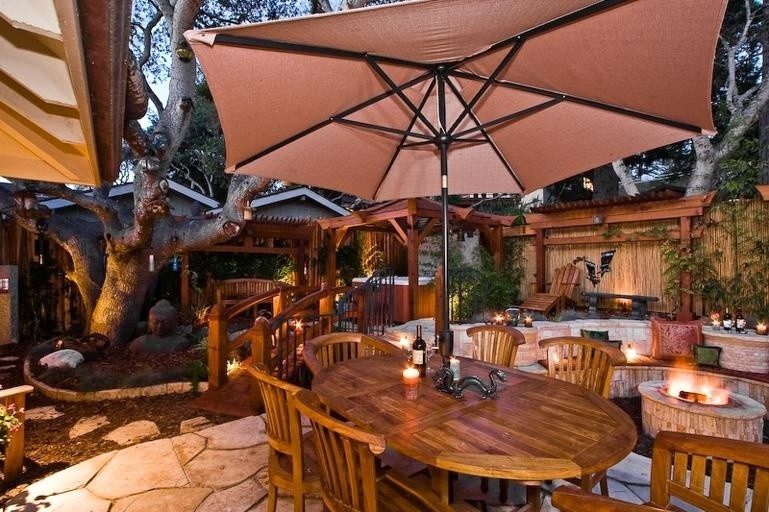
[402,369,420,400]
[449,359,461,381]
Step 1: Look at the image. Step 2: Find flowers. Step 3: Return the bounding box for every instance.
[0,403,25,480]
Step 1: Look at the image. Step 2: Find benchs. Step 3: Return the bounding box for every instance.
[216,278,274,318]
[581,292,659,320]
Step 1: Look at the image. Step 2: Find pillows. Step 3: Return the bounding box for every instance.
[650,319,702,361]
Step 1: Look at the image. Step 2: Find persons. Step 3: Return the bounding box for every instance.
[129,299,192,355]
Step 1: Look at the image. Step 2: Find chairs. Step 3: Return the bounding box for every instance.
[247,325,769,512]
[518,263,580,317]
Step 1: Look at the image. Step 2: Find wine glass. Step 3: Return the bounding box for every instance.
[402,341,413,361]
[427,340,433,371]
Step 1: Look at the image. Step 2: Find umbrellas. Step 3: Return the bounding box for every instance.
[183,0,727,355]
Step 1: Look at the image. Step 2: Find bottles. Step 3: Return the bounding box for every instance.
[735,305,745,334]
[172,255,178,272]
[411,325,427,378]
[722,305,732,331]
[149,254,155,273]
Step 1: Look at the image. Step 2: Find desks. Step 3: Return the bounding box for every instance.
[311,353,638,508]
